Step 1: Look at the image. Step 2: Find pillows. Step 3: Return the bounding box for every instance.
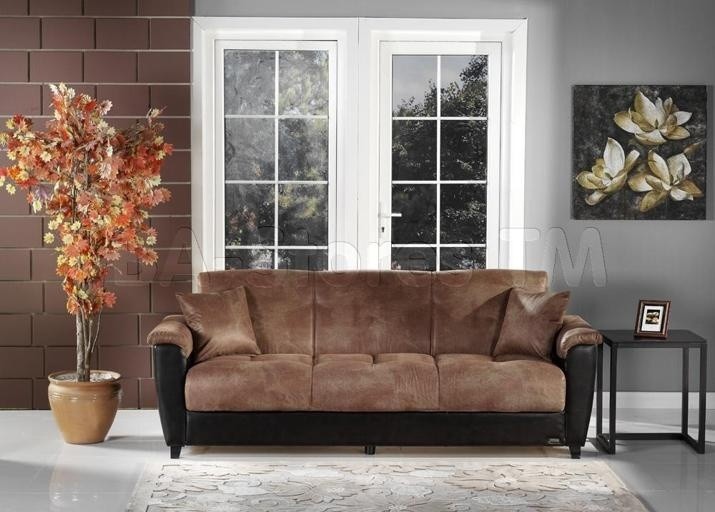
[174,285,261,365]
[493,286,571,362]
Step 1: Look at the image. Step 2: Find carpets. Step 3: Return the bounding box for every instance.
[125,441,651,512]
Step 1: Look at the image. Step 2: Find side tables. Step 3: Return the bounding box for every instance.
[597,329,707,454]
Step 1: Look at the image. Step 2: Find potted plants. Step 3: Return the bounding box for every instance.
[0,81,175,445]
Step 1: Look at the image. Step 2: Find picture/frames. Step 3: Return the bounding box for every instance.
[633,299,671,339]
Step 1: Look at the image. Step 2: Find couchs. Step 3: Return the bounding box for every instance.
[147,268,604,459]
